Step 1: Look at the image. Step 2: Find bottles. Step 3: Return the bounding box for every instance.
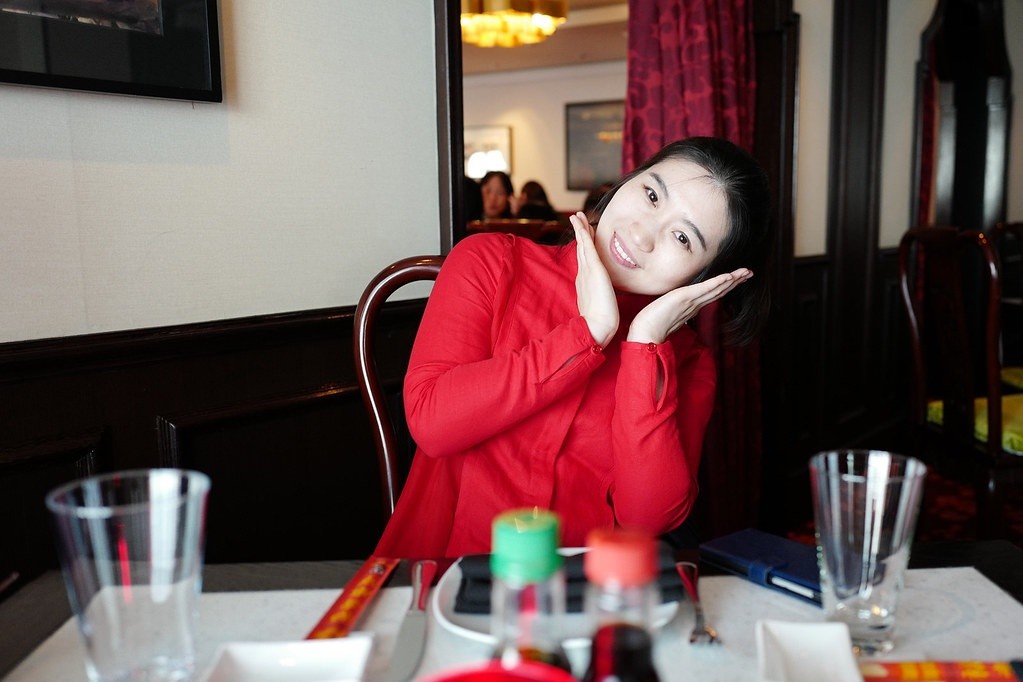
[581,530,663,682]
[485,507,569,673]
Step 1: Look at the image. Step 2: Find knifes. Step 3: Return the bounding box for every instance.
[380,559,438,682]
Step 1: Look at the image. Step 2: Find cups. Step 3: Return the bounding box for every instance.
[809,449,928,659]
[46,467,209,682]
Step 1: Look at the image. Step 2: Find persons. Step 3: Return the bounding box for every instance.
[459,172,557,222]
[373,137,769,557]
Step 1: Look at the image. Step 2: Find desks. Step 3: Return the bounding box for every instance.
[0,555,1023,682]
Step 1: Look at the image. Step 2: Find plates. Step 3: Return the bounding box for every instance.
[432,547,679,651]
[197,634,375,682]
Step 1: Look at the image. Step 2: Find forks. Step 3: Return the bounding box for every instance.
[676,562,719,646]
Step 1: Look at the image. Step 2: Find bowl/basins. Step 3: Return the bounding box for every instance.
[412,659,579,682]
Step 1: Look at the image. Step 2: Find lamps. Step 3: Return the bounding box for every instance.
[461,0,570,51]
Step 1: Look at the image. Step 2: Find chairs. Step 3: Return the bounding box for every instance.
[351,255,449,524]
[896,224,1023,458]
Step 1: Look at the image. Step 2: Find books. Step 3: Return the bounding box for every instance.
[698,529,883,609]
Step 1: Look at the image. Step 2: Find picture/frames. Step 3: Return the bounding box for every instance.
[0,0,223,103]
[563,98,625,191]
